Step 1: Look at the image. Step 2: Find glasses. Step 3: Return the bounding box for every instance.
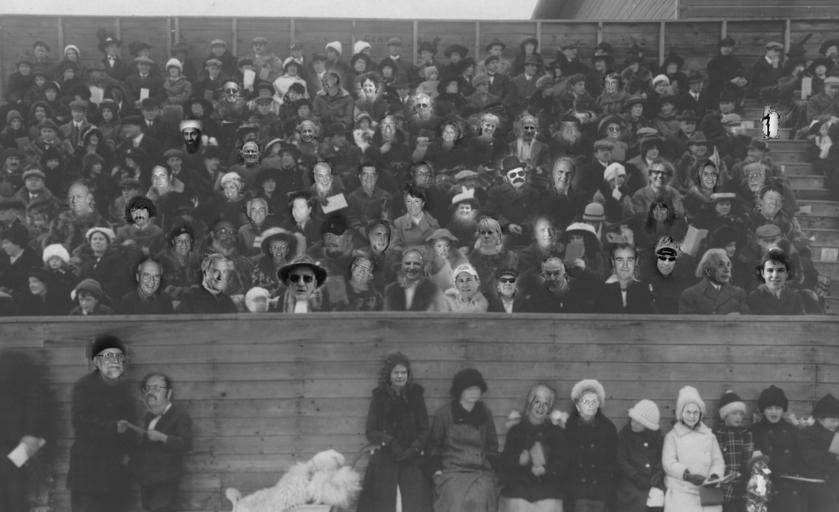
[290,274,313,283]
[142,384,170,392]
[138,272,162,281]
[479,230,497,234]
[415,103,431,109]
[95,352,124,359]
[225,88,238,94]
[658,256,676,262]
[508,171,525,177]
[608,126,621,132]
[500,277,516,284]
[579,398,600,404]
[701,172,718,178]
[651,168,669,175]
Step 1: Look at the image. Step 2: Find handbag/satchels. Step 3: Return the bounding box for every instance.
[645,485,665,508]
[699,483,726,506]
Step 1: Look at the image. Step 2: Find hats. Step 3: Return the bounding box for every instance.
[251,36,268,44]
[85,227,115,245]
[277,255,327,290]
[69,99,88,108]
[637,128,658,138]
[486,39,506,50]
[748,450,770,467]
[520,36,539,46]
[655,235,679,256]
[42,243,70,264]
[259,226,299,252]
[568,377,608,408]
[264,137,284,152]
[6,110,24,124]
[80,126,105,146]
[500,156,528,180]
[64,45,82,60]
[582,203,606,222]
[99,34,122,52]
[134,56,155,65]
[676,385,707,423]
[320,215,349,236]
[710,184,738,199]
[448,187,480,210]
[598,115,625,133]
[91,335,127,357]
[812,393,839,419]
[22,164,47,181]
[626,398,660,431]
[749,139,769,151]
[652,75,670,88]
[453,264,479,282]
[74,278,102,296]
[766,41,783,49]
[495,267,519,279]
[254,97,273,104]
[38,118,58,129]
[689,132,710,144]
[755,223,781,238]
[450,369,488,395]
[325,42,342,55]
[165,58,183,74]
[604,162,626,183]
[425,228,458,243]
[759,385,788,413]
[354,41,372,55]
[418,42,437,54]
[718,389,747,421]
[444,43,469,58]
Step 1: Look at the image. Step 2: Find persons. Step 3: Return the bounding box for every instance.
[0,350,52,511]
[424,369,499,512]
[613,386,838,511]
[134,376,194,512]
[564,378,618,512]
[358,354,431,511]
[497,384,576,512]
[70,334,139,511]
[1,34,838,315]
